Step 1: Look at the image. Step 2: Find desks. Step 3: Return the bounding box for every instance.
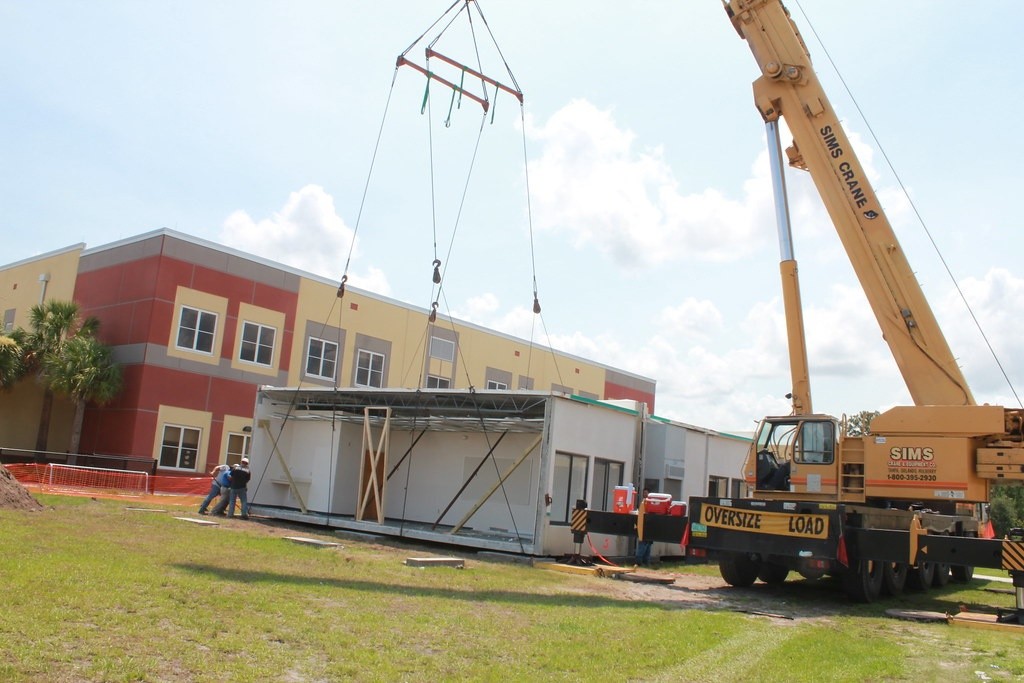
[271,479,310,511]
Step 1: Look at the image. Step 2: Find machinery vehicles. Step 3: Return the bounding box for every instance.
[555,0,1024,626]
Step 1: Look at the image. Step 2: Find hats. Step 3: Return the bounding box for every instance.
[233,463,239,467]
[241,458,249,464]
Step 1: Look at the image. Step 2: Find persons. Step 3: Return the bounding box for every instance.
[767,452,791,491]
[227,458,251,519]
[198,464,240,515]
[632,488,654,567]
[209,463,240,516]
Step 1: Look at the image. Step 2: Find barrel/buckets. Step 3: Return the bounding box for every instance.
[612,486,637,515]
